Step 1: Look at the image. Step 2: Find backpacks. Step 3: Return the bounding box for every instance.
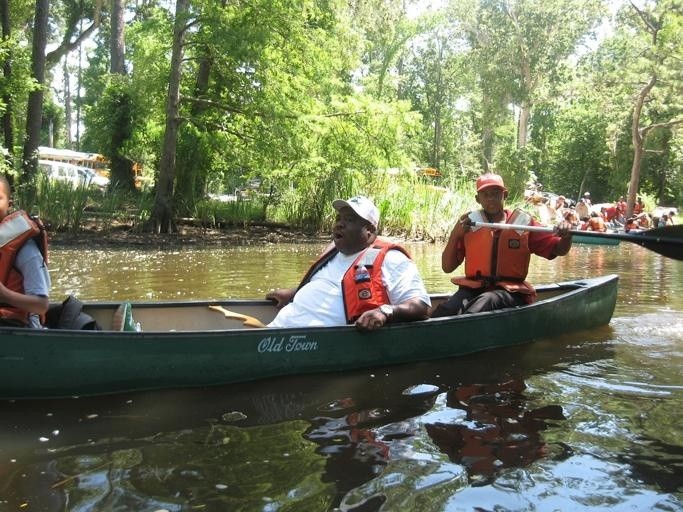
[45,298,101,330]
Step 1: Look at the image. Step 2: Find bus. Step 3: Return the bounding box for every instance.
[37,146,142,193]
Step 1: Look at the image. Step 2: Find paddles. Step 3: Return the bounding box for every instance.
[468,220,683,262]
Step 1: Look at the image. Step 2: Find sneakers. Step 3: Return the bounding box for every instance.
[112,302,142,331]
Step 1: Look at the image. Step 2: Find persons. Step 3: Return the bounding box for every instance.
[0,172,52,332]
[428,172,574,318]
[529,182,678,234]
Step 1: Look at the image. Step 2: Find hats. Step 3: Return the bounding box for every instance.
[333,196,381,230]
[477,173,507,193]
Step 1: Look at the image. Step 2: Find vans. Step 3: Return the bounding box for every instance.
[37,161,93,190]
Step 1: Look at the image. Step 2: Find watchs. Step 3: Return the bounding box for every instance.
[378,303,395,324]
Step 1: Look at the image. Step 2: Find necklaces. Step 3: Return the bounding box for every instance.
[263,192,435,335]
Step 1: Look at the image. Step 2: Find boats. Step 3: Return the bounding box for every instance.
[26,324,619,444]
[0,273,619,401]
[616,227,642,235]
[569,235,621,247]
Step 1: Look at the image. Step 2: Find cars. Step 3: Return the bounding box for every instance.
[235,179,280,204]
[83,167,111,186]
[541,192,575,208]
[416,167,442,176]
